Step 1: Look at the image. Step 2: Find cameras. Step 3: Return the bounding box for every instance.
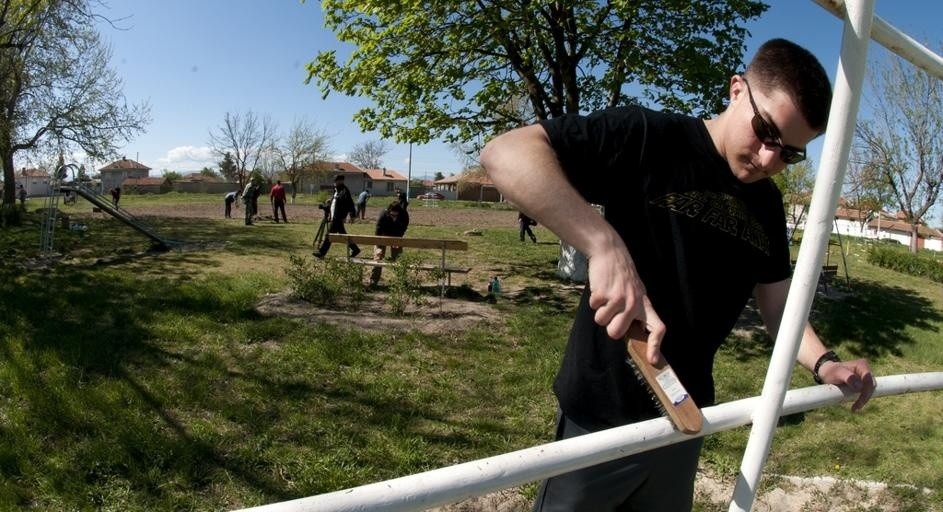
[319,204,331,213]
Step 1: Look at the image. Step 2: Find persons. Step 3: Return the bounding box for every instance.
[398,193,408,211]
[370,201,406,279]
[270,180,287,223]
[109,188,121,209]
[223,190,241,218]
[19,185,26,205]
[474,37,878,512]
[311,174,361,259]
[353,189,370,220]
[241,177,258,225]
[517,211,536,243]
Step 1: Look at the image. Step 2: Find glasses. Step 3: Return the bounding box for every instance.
[741,78,805,165]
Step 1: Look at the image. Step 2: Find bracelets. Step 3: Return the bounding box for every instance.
[813,351,840,383]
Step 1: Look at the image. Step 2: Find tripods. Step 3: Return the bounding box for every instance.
[311,213,329,249]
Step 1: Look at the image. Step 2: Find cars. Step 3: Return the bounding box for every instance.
[416,191,445,200]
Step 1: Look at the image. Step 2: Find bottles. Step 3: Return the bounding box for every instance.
[487,276,500,292]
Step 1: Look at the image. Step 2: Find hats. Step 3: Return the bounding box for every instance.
[333,174,345,180]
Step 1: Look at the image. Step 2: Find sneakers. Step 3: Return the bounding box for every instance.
[350,249,361,257]
[313,252,324,259]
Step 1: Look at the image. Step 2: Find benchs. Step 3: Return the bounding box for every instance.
[328,233,472,299]
[791,260,838,295]
